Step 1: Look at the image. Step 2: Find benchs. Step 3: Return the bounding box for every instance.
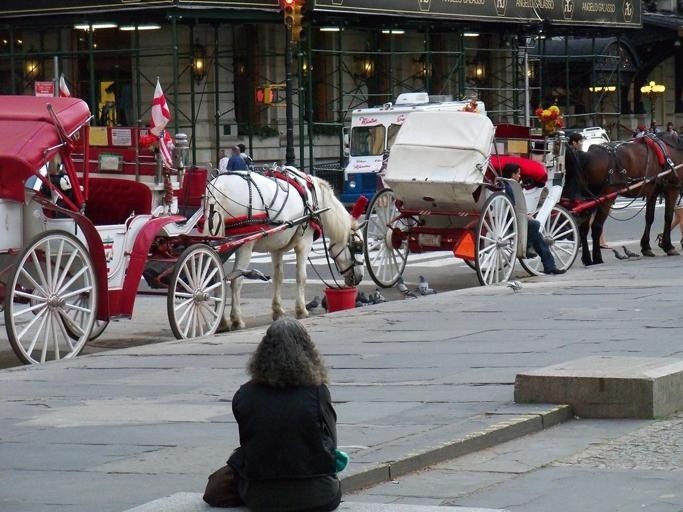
[59,177,154,226]
[21,186,92,255]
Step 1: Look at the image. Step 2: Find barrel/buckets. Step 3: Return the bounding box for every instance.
[324,287,359,313]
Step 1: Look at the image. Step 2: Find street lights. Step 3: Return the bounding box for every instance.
[640,81,665,134]
[589,86,616,129]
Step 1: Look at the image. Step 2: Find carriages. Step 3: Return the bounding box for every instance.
[0,95,368,366]
[363,112,683,289]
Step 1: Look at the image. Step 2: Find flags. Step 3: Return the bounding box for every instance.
[150,80,171,137]
[159,130,174,168]
[59,76,72,97]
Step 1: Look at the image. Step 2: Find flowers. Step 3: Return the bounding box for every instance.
[536,105,564,137]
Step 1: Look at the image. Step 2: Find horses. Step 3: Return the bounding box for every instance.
[578,131,683,266]
[206,166,368,334]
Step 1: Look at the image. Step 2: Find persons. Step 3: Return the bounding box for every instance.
[632,119,677,136]
[218,144,254,175]
[562,134,586,197]
[592,211,612,250]
[227,318,342,511]
[654,194,683,250]
[503,163,567,275]
[40,163,76,218]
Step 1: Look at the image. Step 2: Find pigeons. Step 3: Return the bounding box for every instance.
[322,295,327,313]
[612,250,628,260]
[223,269,272,282]
[507,281,522,295]
[356,288,388,308]
[396,276,440,300]
[306,296,320,314]
[622,246,639,259]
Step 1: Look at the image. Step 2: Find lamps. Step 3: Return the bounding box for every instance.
[190,36,208,86]
[299,39,310,78]
[526,62,537,82]
[423,61,433,78]
[475,60,487,83]
[22,43,43,89]
[360,55,375,80]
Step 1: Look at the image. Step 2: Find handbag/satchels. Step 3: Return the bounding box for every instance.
[202,464,246,507]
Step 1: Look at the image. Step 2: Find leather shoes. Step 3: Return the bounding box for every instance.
[544,266,565,275]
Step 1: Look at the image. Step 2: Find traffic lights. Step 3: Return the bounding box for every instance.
[284,0,306,42]
[255,87,279,104]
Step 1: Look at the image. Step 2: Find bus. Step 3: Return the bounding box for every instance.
[340,92,485,207]
